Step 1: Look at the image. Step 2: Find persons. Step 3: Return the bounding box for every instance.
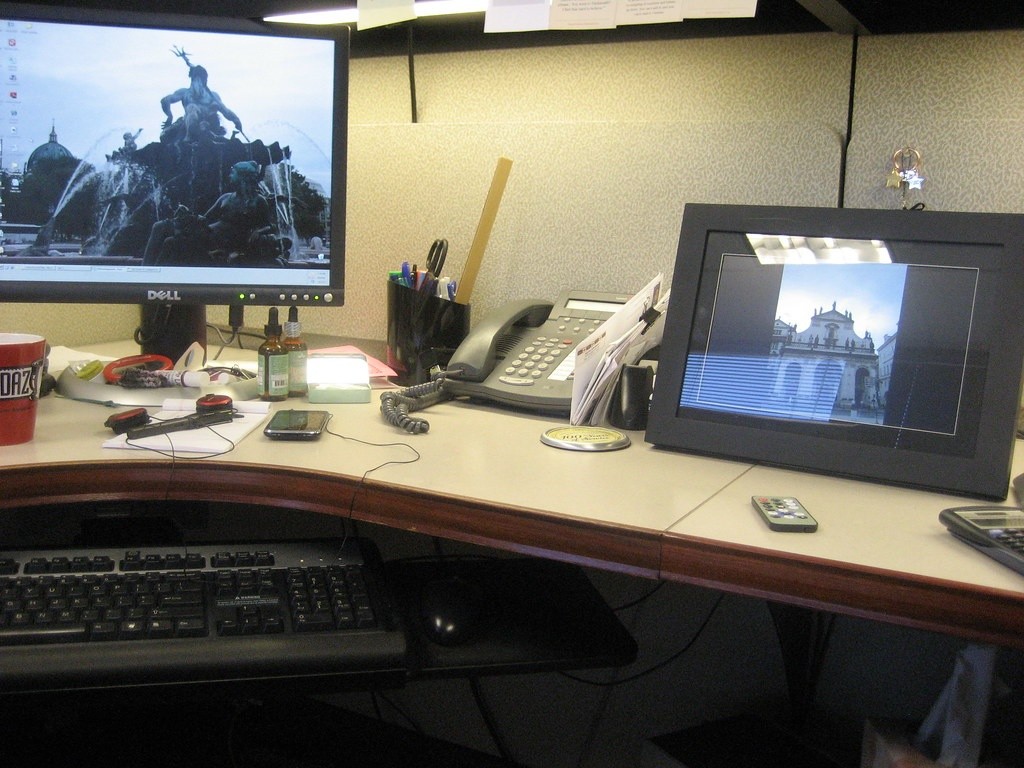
[159,64,244,146]
[114,127,146,159]
[137,158,277,265]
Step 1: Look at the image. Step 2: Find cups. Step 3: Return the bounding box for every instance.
[0,331,46,447]
[384,279,471,388]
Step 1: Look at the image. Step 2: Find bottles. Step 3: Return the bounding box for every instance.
[280,307,310,397]
[256,307,289,401]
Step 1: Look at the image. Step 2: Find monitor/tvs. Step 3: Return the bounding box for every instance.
[1,1,346,307]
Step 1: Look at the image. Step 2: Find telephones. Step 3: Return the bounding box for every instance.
[441,289,639,414]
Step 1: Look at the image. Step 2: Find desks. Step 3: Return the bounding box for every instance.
[1,326,1023,647]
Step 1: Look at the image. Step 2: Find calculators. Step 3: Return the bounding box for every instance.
[939,504,1024,576]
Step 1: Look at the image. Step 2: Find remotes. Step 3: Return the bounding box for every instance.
[752,494,818,533]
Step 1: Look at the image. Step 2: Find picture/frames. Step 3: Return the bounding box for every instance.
[644,203,1023,499]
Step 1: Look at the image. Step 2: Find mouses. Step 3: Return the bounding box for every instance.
[417,575,470,648]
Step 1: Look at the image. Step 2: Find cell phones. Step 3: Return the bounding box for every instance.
[265,410,328,440]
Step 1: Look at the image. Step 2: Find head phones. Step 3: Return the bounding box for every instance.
[103,393,232,434]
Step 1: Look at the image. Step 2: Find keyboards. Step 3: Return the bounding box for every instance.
[0,536,400,678]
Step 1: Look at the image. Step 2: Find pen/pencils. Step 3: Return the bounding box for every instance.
[387,238,456,303]
